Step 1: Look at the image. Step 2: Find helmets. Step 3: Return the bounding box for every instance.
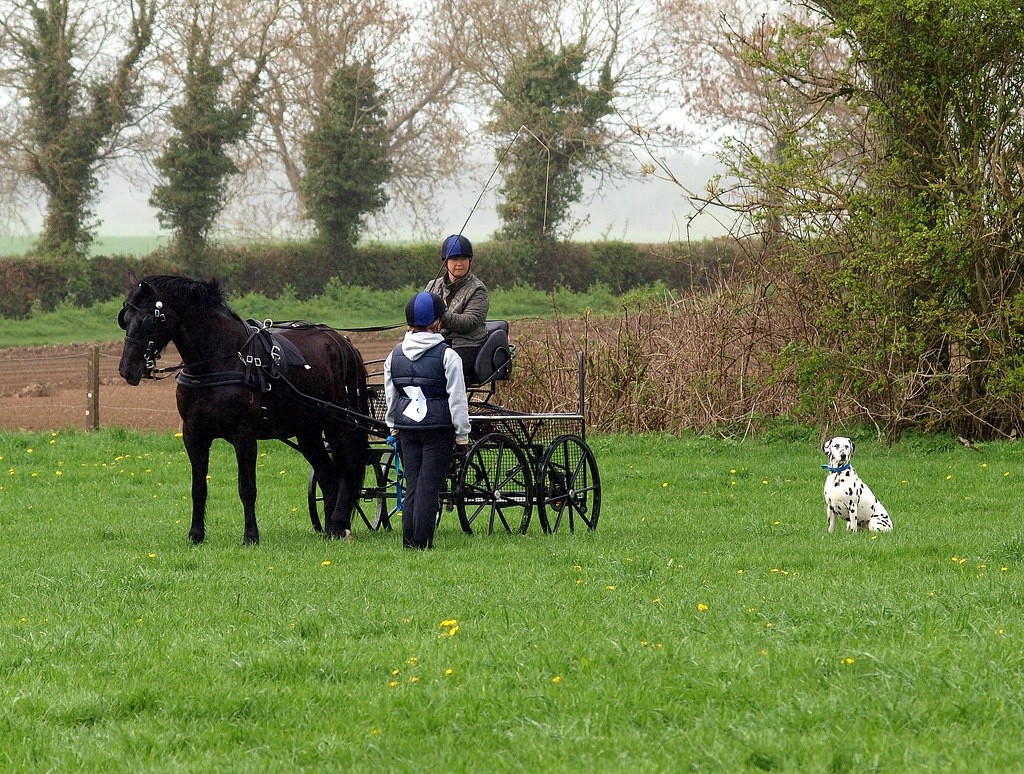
[405,291,446,327]
[442,234,474,261]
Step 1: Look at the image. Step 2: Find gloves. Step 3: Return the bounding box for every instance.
[450,444,468,459]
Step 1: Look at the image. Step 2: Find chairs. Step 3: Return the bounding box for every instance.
[471,320,513,383]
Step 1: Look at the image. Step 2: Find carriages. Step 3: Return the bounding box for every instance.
[117,276,602,543]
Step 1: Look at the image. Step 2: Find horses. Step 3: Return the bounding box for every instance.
[117,274,372,546]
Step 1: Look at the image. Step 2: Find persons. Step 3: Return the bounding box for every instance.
[426,234,489,391]
[382,291,470,551]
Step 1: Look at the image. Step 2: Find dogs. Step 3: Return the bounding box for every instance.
[821,436,895,537]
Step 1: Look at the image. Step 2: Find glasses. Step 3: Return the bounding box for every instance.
[448,256,471,262]
